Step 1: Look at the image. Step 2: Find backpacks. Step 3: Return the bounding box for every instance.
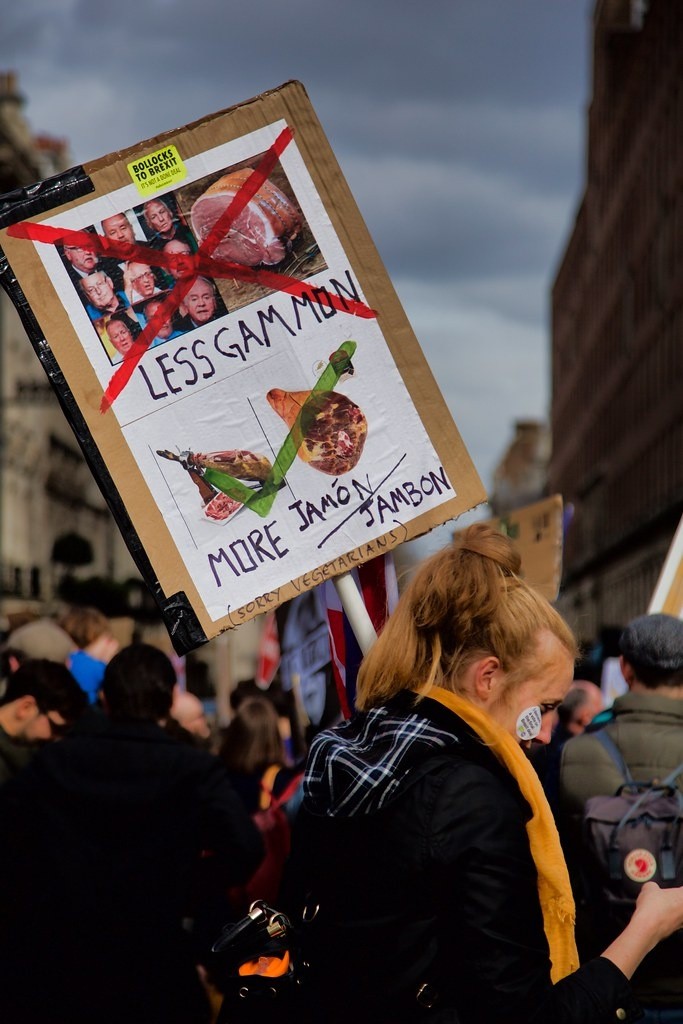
[583,729,683,903]
[227,768,307,919]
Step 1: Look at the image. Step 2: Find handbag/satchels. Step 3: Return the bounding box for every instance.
[215,900,344,1024]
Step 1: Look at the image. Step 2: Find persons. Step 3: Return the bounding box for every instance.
[61,199,216,363]
[275,522,683,1024]
[0,607,683,1024]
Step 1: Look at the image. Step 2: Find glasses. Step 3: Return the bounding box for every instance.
[45,714,66,738]
